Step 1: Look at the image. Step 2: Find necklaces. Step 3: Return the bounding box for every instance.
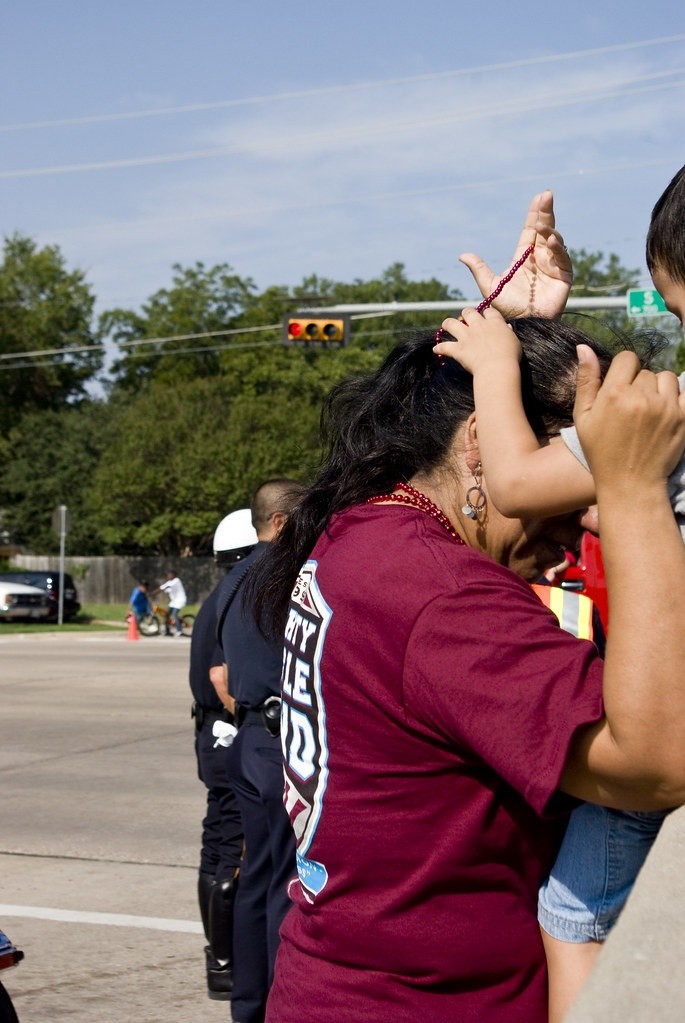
[363,482,467,546]
[436,241,535,367]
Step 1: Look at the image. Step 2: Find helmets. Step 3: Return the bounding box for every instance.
[212,509,257,567]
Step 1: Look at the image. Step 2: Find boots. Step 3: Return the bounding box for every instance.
[207,882,234,1000]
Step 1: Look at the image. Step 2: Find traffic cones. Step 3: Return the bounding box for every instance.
[125,612,140,641]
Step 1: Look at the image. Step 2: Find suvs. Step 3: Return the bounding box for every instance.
[1,569,79,624]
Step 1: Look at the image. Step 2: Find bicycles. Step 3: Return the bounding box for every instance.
[138,593,196,638]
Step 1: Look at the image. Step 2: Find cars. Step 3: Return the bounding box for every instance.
[0,580,51,622]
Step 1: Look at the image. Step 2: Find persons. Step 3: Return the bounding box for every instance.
[259,188,685,1023]
[429,165,685,1022]
[189,508,259,1000]
[130,569,188,638]
[214,479,310,1023]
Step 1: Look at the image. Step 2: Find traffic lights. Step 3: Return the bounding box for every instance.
[287,319,344,343]
